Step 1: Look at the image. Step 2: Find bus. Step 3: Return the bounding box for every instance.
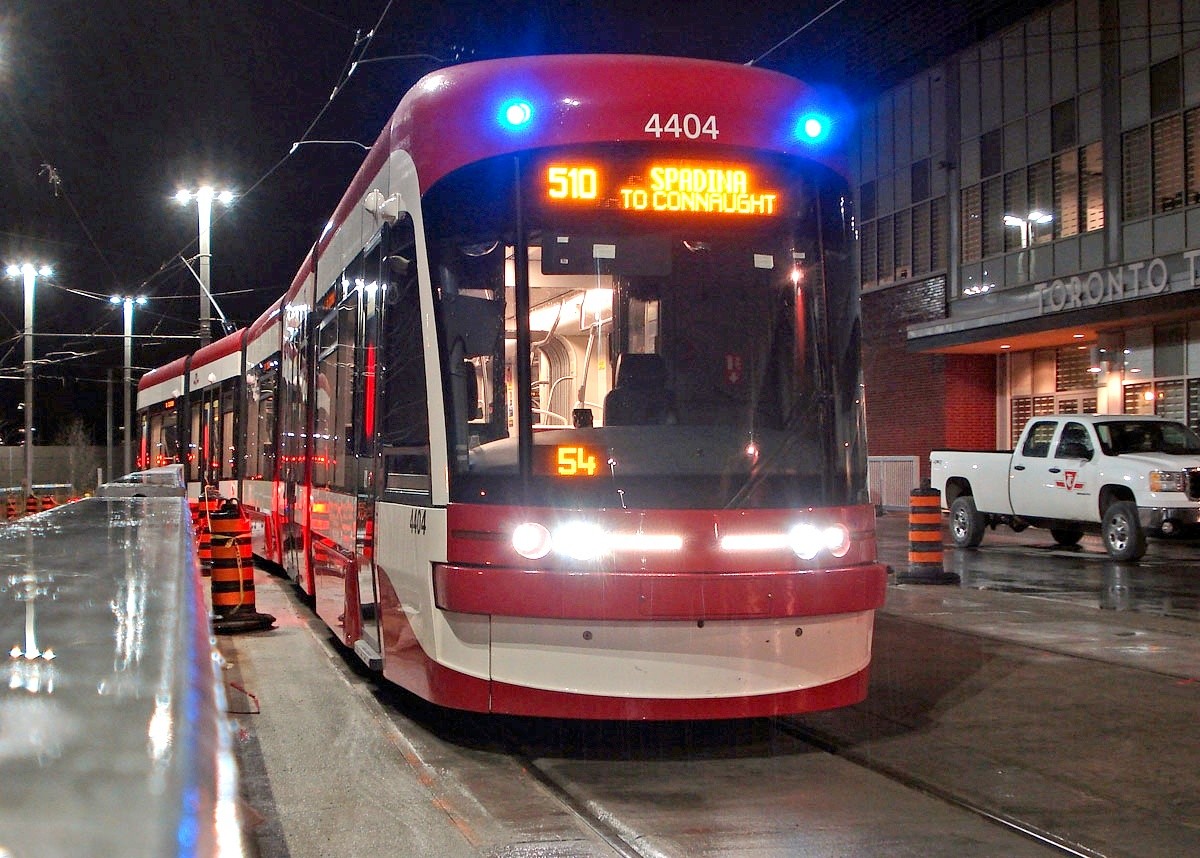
[133,52,891,729]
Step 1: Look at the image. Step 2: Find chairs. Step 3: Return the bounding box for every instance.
[603,352,676,426]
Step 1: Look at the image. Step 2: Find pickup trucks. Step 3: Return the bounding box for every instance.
[928,413,1200,563]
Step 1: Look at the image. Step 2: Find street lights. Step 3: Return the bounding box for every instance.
[110,293,149,481]
[175,189,237,352]
[1003,210,1054,282]
[7,262,52,495]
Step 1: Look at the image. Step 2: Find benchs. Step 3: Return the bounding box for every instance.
[531,403,602,432]
